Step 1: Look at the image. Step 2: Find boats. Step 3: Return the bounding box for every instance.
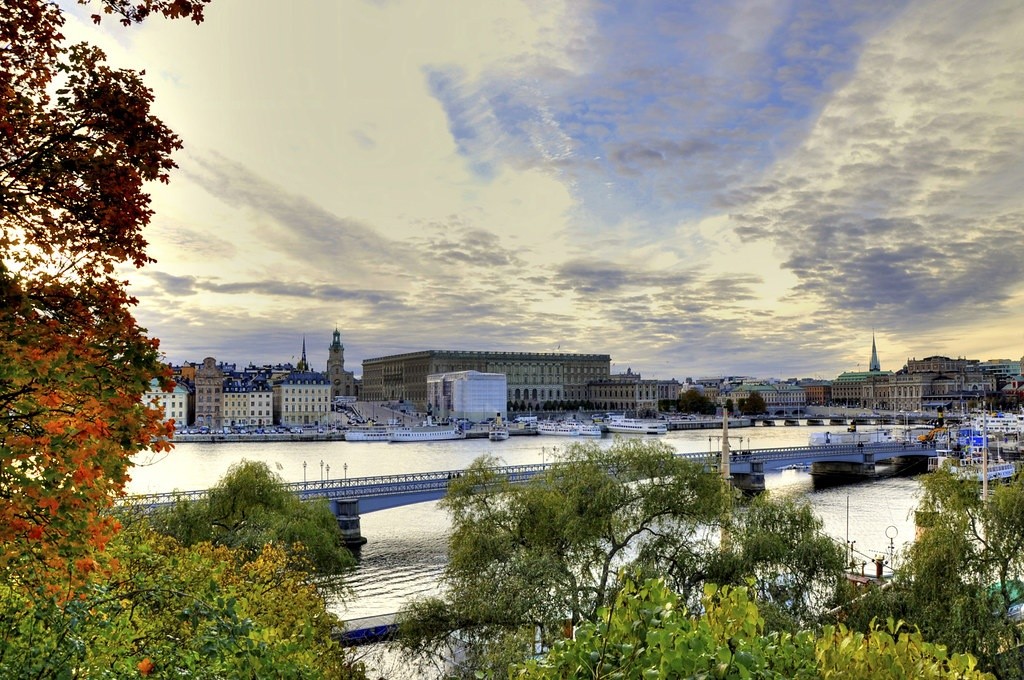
[927,444,1018,484]
[536,422,581,437]
[386,405,466,442]
[566,421,603,440]
[488,415,510,441]
[606,418,669,435]
[344,411,412,441]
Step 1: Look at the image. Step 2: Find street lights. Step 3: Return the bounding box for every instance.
[303,461,308,480]
[326,464,330,481]
[541,446,546,472]
[553,446,559,467]
[343,463,348,479]
[320,460,325,481]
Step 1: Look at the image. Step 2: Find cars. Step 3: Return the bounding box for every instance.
[189,430,194,434]
[181,430,188,434]
[175,430,181,435]
[196,425,304,435]
[317,427,323,433]
[336,406,363,430]
